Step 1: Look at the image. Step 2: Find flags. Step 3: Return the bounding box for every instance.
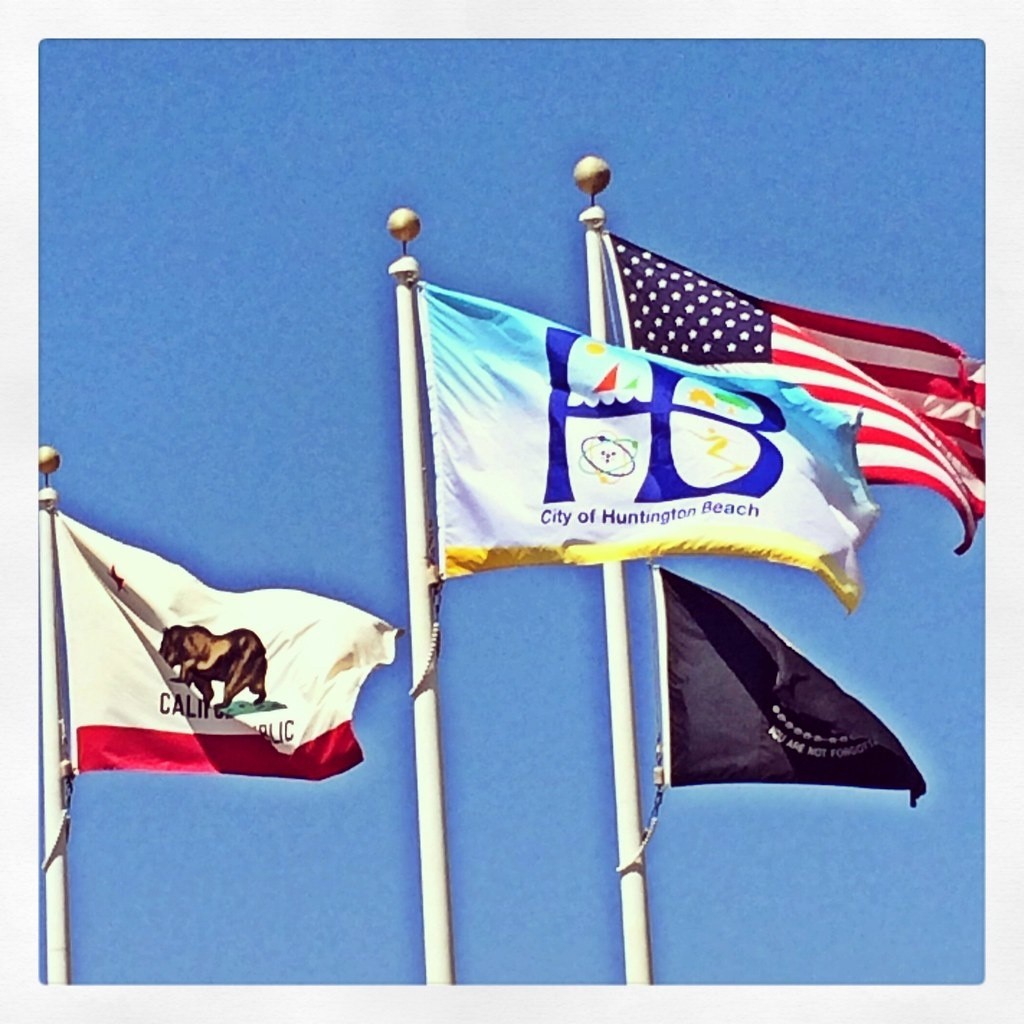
[606,232,985,557]
[54,511,405,781]
[410,283,880,615]
[652,566,926,808]
